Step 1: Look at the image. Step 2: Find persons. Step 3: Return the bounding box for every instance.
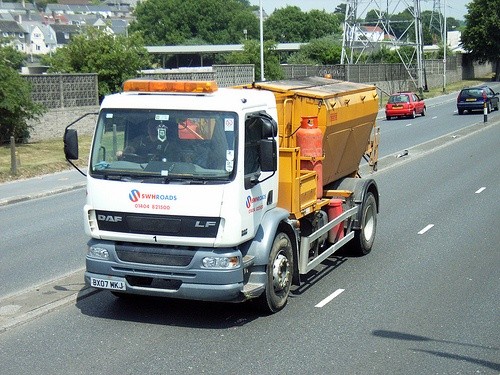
[116,120,178,162]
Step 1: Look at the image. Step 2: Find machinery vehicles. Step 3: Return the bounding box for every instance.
[62,75,383,313]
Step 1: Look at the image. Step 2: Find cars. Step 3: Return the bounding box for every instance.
[384,91,427,121]
[455,86,499,115]
